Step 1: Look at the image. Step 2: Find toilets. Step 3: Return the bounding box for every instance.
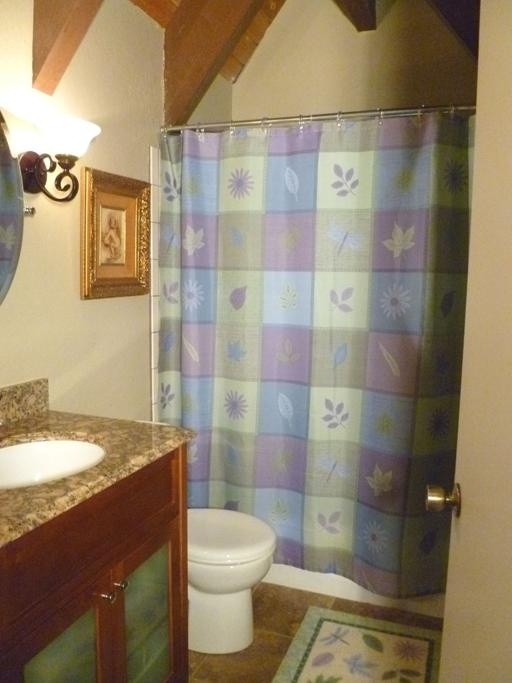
[187,508,277,655]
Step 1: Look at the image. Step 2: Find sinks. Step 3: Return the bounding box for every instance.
[0,430,108,490]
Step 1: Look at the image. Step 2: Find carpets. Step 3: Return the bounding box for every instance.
[270,605,442,683]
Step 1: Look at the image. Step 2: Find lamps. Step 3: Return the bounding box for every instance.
[17,118,101,202]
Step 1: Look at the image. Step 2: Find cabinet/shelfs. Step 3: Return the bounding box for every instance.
[0,443,188,683]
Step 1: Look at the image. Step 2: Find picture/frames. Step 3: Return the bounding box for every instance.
[80,166,151,300]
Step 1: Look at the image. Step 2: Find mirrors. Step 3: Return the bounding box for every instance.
[0,109,24,307]
